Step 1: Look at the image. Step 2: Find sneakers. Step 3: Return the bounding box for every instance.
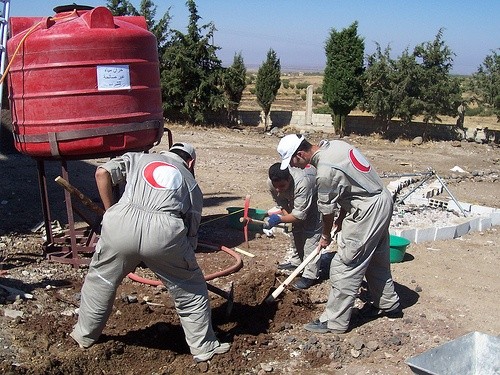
[193,342,231,362]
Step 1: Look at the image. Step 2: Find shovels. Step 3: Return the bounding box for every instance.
[55,176,234,314]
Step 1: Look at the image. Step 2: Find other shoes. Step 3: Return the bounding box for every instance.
[305,320,347,334]
[283,262,301,275]
[292,277,319,289]
[362,300,401,316]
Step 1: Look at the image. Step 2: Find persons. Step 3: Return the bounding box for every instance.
[276,134,401,334]
[69,142,231,362]
[261,162,321,290]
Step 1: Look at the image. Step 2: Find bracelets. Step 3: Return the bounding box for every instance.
[322,235,331,240]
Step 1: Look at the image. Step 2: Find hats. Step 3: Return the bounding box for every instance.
[169,142,196,179]
[276,134,305,171]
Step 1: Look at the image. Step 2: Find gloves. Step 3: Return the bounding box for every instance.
[268,215,282,230]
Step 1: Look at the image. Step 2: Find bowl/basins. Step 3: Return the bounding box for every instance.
[226,207,268,230]
[389,234,410,263]
